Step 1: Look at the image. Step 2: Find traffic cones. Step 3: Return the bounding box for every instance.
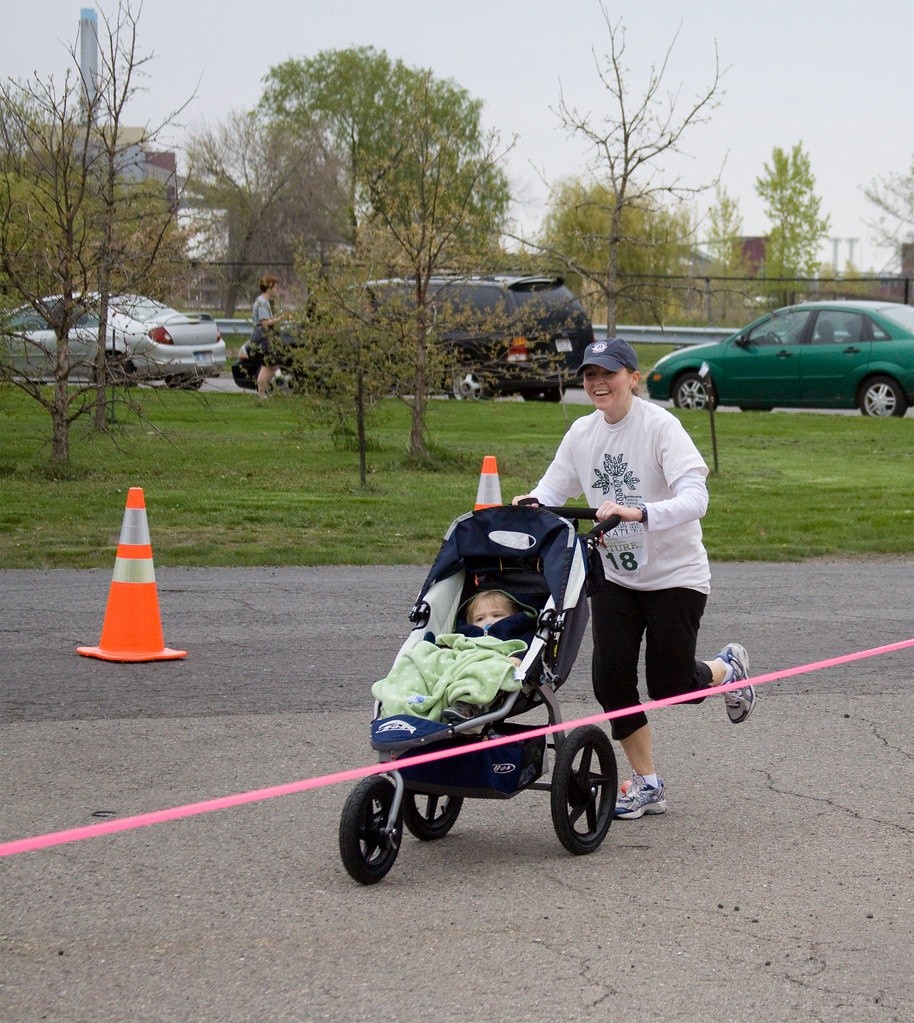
[472,456,504,511]
[77,486,187,661]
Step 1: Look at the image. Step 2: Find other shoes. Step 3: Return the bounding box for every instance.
[443,705,476,722]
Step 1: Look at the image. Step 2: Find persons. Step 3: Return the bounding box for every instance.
[434,591,539,726]
[512,338,756,819]
[250,275,281,397]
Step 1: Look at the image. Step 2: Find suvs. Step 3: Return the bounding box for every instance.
[230,270,594,402]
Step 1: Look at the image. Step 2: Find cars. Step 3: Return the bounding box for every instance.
[0,291,227,390]
[646,299,914,418]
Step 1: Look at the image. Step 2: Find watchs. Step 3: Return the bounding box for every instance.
[635,504,647,523]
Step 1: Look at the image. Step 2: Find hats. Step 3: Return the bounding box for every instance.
[576,337,638,378]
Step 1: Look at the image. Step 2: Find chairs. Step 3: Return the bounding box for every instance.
[809,318,863,344]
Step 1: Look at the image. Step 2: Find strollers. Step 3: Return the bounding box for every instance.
[339,496,617,886]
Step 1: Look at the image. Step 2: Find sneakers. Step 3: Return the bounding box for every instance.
[614,770,668,819]
[714,643,757,723]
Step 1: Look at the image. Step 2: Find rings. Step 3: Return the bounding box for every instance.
[602,511,606,515]
